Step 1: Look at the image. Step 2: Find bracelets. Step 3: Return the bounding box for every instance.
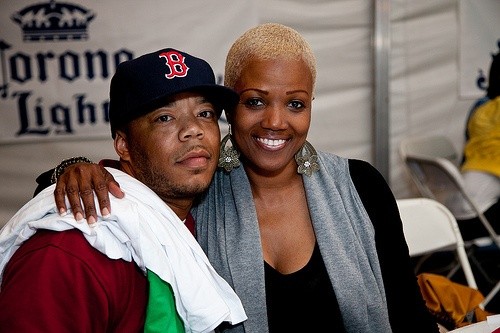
[51,155,92,185]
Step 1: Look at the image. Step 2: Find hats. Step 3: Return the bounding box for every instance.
[110,48,241,140]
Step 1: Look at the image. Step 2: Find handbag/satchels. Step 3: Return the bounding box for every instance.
[417,273,487,331]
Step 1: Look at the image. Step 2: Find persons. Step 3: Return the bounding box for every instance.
[33,23,439,333]
[453,97,500,283]
[0,47,241,333]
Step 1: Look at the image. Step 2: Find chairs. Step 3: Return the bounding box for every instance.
[394,134,500,333]
